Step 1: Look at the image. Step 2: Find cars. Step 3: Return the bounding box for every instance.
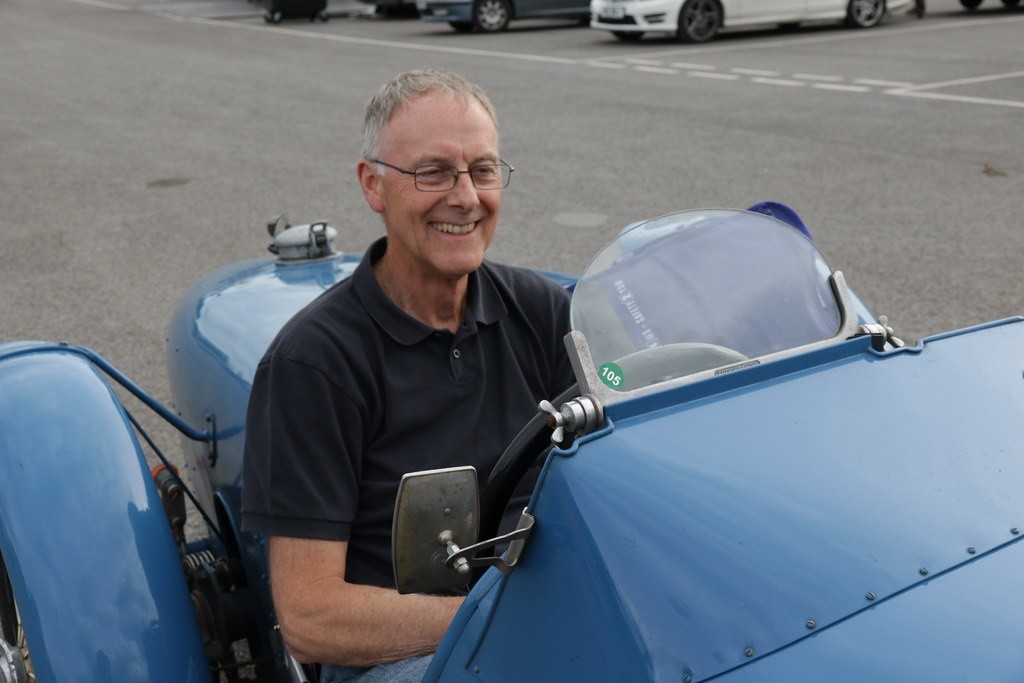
[413,0,916,45]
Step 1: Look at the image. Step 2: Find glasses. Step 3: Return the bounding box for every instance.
[371,157,516,192]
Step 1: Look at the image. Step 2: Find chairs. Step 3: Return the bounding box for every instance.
[562,202,877,384]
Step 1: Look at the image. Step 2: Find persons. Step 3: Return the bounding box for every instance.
[240,71,592,683]
